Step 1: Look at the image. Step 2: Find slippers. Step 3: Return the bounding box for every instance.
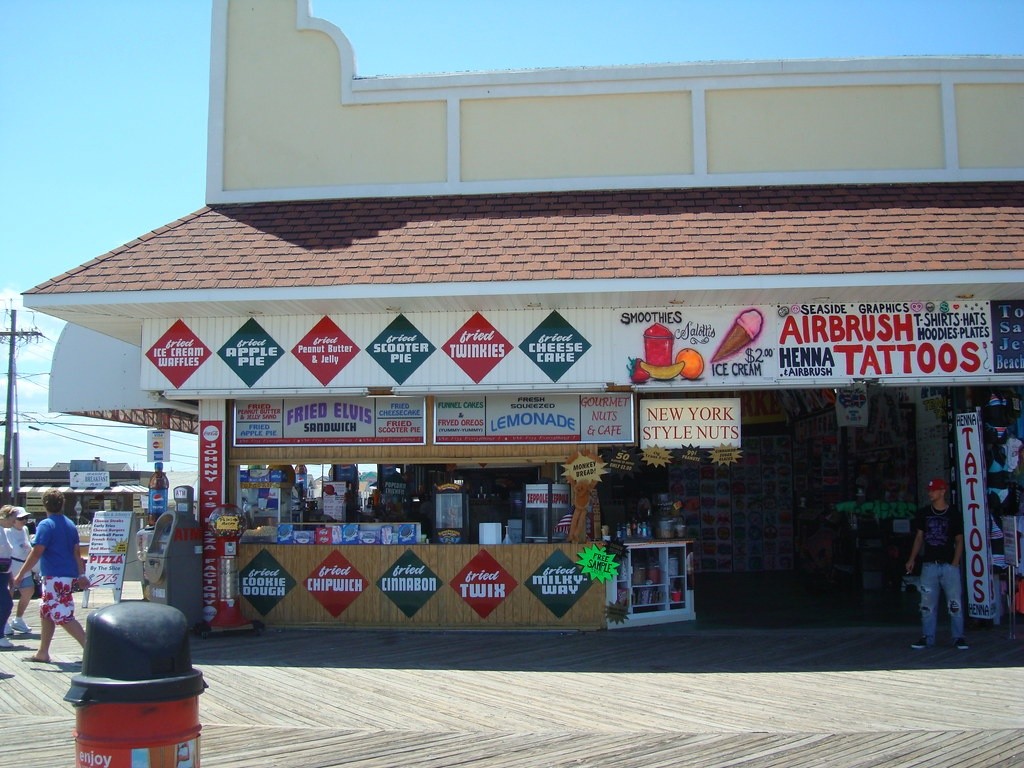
[23,655,52,663]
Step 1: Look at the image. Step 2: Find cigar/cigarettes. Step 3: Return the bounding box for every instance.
[906,571,909,574]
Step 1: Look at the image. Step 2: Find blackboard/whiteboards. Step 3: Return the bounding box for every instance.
[85,517,132,588]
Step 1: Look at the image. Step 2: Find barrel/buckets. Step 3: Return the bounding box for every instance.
[632,562,646,584]
[668,554,679,576]
[654,517,676,539]
[646,561,661,584]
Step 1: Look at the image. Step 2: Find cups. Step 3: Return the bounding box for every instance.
[671,590,682,602]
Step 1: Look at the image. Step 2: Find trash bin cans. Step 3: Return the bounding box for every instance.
[63,601,206,768]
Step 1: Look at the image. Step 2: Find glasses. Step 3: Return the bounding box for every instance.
[16,516,28,521]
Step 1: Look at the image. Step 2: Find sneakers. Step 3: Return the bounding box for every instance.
[910,638,927,648]
[11,619,32,633]
[3,621,14,635]
[953,638,969,649]
[0,637,13,648]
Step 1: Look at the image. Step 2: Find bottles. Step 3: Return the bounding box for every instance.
[616,519,652,538]
[148,461,169,526]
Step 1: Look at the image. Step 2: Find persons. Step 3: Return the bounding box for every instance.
[3,507,35,636]
[904,477,971,652]
[0,505,20,651]
[12,488,90,664]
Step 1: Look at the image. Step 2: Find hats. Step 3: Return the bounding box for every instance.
[14,506,31,519]
[927,479,947,491]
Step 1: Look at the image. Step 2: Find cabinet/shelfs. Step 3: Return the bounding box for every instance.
[606,537,696,631]
